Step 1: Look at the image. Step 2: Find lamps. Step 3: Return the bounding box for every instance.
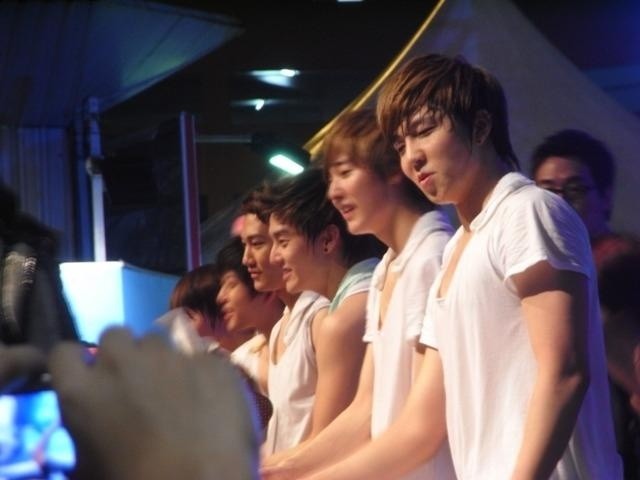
[266,141,310,176]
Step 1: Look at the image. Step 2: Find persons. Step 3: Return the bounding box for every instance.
[41,317,265,480]
[251,106,460,478]
[525,129,640,479]
[169,261,263,376]
[213,235,285,400]
[236,179,334,468]
[255,165,385,475]
[301,49,628,480]
[0,178,83,382]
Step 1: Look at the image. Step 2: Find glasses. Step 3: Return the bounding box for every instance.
[545,187,605,201]
